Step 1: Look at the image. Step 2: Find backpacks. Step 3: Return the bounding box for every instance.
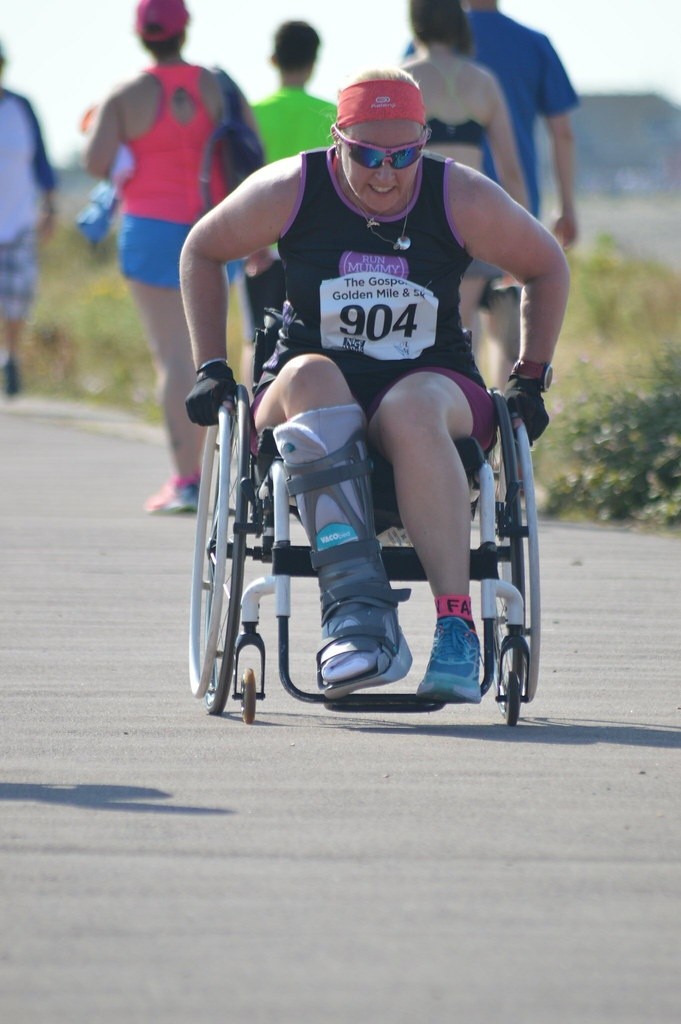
[199,66,264,211]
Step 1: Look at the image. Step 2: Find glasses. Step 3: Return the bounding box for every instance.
[334,126,427,169]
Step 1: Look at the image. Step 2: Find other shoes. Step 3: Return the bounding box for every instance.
[0,356,18,394]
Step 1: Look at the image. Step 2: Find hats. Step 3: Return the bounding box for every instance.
[135,0,190,42]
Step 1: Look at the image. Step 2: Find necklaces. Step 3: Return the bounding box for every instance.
[340,174,410,251]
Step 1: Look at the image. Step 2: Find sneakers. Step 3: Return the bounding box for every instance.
[144,468,200,513]
[417,616,481,704]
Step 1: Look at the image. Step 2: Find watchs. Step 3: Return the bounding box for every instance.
[512,360,553,393]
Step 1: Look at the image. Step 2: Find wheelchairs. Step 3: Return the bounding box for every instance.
[190,308,541,724]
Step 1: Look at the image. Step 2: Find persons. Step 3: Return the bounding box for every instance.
[179,68,569,702]
[399,0,581,392]
[83,0,271,513]
[240,22,340,408]
[394,0,528,329]
[0,44,54,400]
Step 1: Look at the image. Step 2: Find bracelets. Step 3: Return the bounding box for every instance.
[199,359,228,369]
